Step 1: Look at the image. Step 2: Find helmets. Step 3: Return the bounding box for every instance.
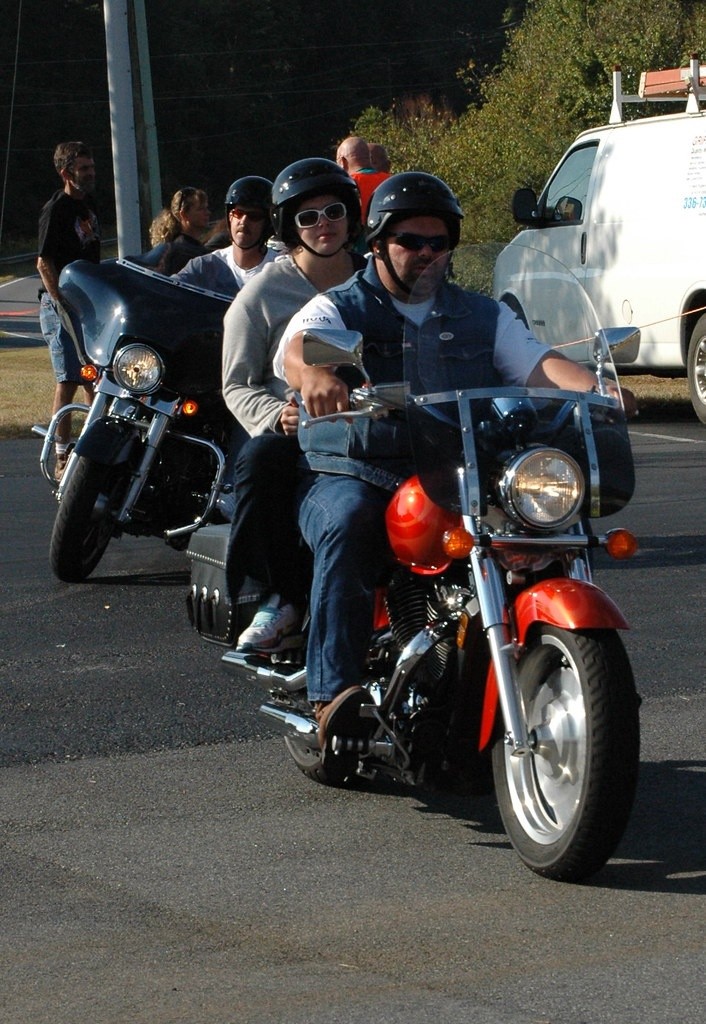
[366,171,464,250]
[224,176,274,214]
[270,157,362,244]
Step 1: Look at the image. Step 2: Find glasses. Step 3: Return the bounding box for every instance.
[386,231,453,252]
[230,209,268,221]
[294,202,349,228]
[179,187,194,210]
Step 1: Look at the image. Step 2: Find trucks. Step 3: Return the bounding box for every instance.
[490,59,706,423]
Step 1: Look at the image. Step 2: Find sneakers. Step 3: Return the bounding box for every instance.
[235,593,298,652]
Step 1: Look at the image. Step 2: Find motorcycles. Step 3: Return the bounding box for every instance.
[30,243,233,582]
[186,241,641,883]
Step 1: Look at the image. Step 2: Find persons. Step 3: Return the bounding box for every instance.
[146,135,392,514]
[36,140,101,480]
[221,157,368,659]
[272,172,638,749]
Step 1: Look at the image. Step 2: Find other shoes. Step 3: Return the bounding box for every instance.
[54,453,66,479]
[316,685,376,773]
